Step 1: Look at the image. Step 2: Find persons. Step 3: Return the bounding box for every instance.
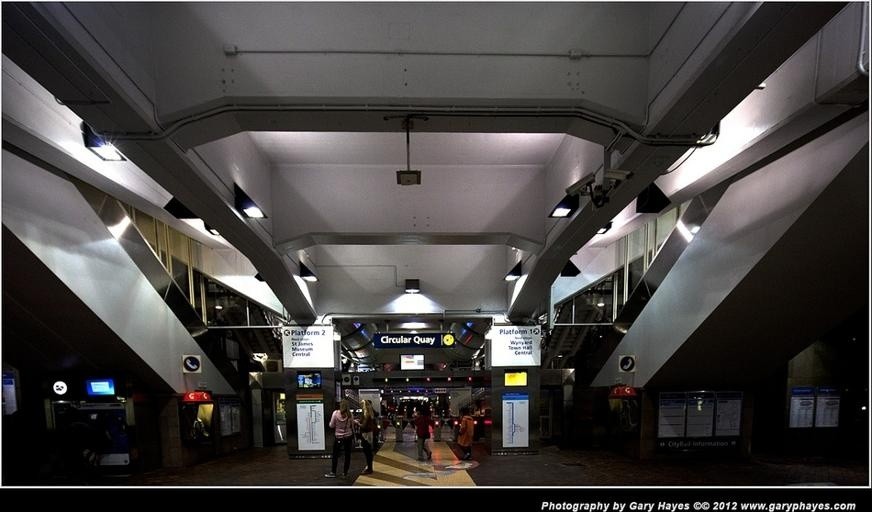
[457,406,475,460]
[410,409,434,462]
[353,398,377,474]
[324,399,356,478]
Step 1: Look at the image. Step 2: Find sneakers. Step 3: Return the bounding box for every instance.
[324,472,336,477]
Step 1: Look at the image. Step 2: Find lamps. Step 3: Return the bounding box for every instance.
[503,260,525,286]
[399,275,426,296]
[295,260,320,283]
[230,179,268,223]
[544,190,580,221]
[77,123,126,164]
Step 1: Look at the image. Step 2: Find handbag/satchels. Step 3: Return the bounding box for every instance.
[334,438,344,447]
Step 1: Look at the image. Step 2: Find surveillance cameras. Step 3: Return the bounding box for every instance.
[605,169,633,181]
[566,172,595,196]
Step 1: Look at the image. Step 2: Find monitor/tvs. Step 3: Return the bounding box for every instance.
[400,353,425,371]
[297,371,322,389]
[504,372,528,386]
[85,378,115,402]
